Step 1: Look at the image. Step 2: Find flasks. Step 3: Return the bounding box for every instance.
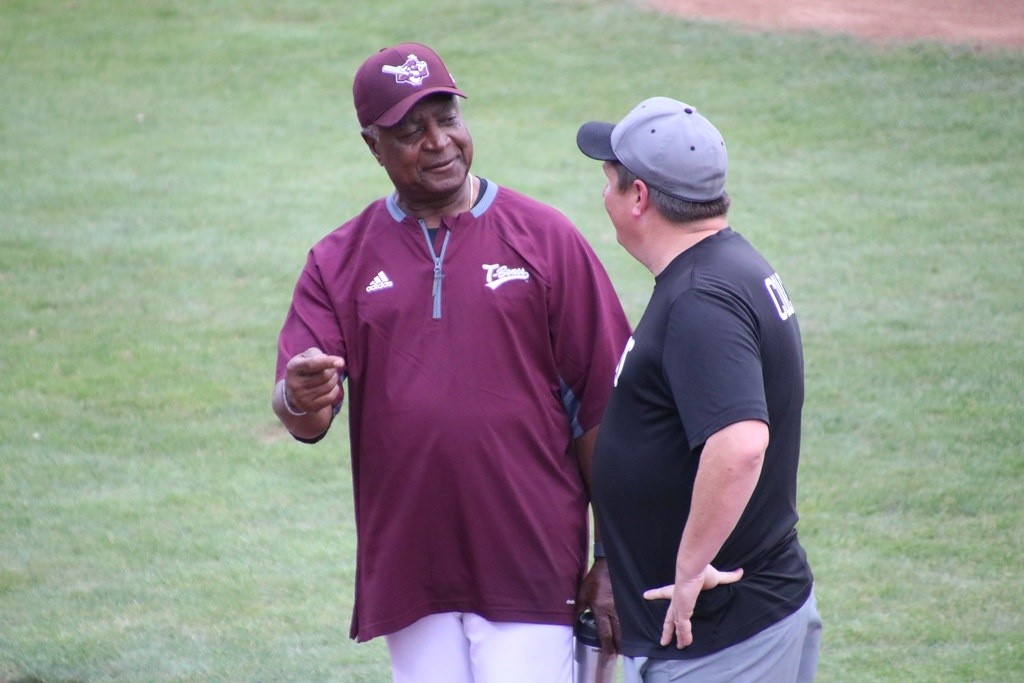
[574,612,618,683]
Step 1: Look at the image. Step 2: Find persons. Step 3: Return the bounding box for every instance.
[272,42,632,682]
[577,97,821,682]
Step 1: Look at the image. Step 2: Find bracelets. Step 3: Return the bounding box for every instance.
[593,543,606,558]
[282,382,306,416]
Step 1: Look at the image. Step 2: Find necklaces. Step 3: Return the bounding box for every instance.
[468,173,474,209]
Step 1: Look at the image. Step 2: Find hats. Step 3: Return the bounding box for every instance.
[576,97,727,202]
[352,43,467,128]
